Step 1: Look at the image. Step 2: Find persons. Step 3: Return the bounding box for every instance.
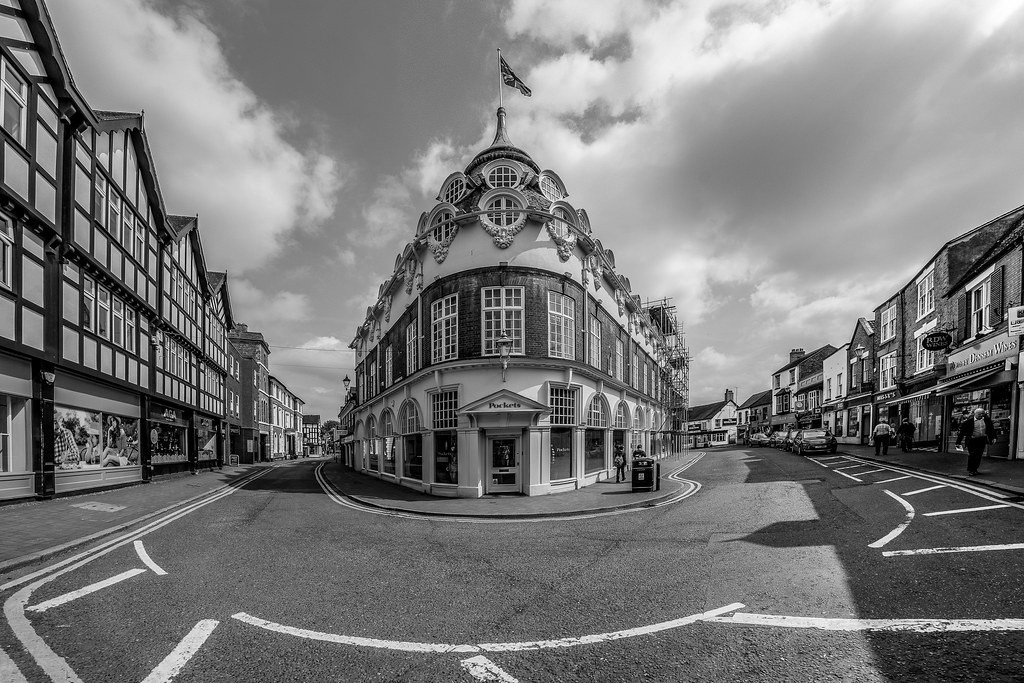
[960,410,970,424]
[55,411,101,466]
[895,417,916,452]
[956,408,997,476]
[163,408,177,422]
[871,417,892,456]
[633,444,646,461]
[103,416,139,467]
[891,421,897,445]
[994,412,1007,431]
[201,419,210,429]
[614,445,626,484]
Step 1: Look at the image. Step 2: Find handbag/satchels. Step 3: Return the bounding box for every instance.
[868,438,875,446]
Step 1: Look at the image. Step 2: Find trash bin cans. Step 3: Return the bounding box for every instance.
[632,457,655,492]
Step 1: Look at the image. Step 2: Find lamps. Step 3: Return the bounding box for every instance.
[496,329,513,369]
[343,374,351,390]
[854,344,912,361]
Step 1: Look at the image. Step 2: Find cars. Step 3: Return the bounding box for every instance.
[782,429,802,452]
[769,431,789,448]
[748,433,769,447]
[588,445,603,457]
[791,428,837,455]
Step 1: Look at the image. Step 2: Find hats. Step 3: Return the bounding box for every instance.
[879,416,888,422]
[107,416,121,425]
[961,410,970,414]
[890,423,896,427]
[902,418,908,422]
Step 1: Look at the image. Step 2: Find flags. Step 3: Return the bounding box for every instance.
[499,51,533,96]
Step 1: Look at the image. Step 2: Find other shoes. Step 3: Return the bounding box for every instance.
[622,478,626,481]
[616,481,620,483]
[968,471,982,476]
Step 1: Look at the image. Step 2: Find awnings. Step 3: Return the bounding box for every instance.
[886,363,1004,406]
[825,388,873,406]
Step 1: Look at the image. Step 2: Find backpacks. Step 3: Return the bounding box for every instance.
[634,450,644,460]
[613,454,624,467]
